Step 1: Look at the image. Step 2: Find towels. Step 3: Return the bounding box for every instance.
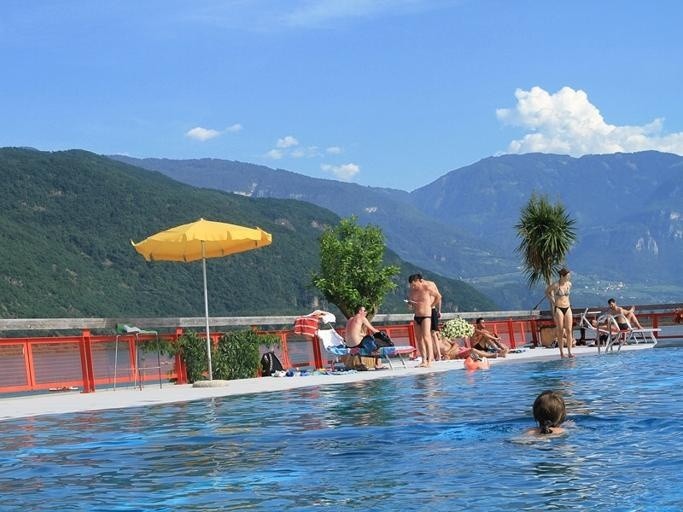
[292,308,337,337]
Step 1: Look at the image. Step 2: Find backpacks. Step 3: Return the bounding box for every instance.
[261,352,283,376]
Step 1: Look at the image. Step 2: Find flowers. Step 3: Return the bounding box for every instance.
[439,316,477,341]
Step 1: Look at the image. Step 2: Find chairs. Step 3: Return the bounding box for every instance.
[316,324,415,370]
[577,305,663,354]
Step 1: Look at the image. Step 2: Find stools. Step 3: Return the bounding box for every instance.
[112,323,162,392]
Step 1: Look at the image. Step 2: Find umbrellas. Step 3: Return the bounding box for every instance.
[129,218,272,381]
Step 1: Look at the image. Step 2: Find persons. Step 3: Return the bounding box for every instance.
[608,299,623,321]
[408,275,443,368]
[344,304,389,371]
[433,330,507,359]
[470,318,509,353]
[522,390,578,436]
[588,305,644,333]
[546,268,575,358]
[416,273,442,362]
